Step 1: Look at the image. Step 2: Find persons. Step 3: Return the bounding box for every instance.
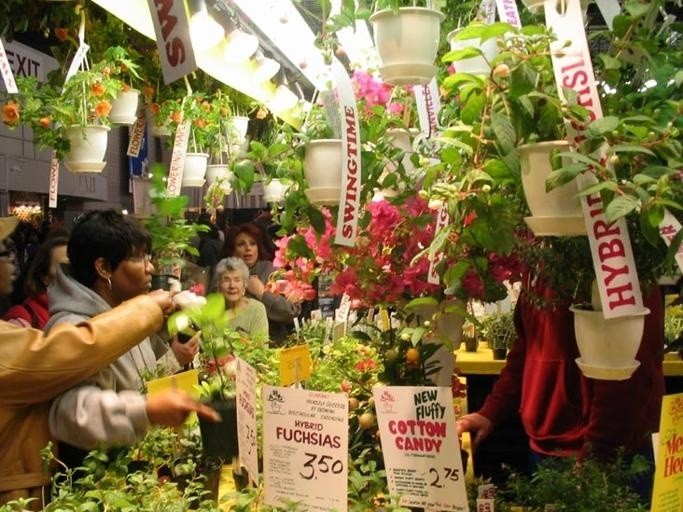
[454,235,664,511]
[0,211,302,511]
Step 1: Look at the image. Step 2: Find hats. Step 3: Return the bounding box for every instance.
[0,215,16,240]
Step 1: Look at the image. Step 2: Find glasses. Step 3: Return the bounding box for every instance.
[0,237,17,258]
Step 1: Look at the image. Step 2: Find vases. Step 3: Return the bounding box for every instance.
[568,303,652,382]
[366,7,446,85]
[517,139,586,237]
[53,124,110,175]
[180,153,231,187]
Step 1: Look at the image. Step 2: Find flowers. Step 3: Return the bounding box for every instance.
[0,8,250,165]
